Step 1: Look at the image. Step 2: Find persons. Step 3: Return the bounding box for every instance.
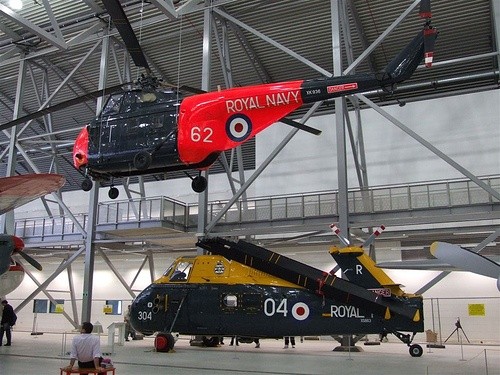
[0,299,14,346]
[61,322,107,375]
[219,337,224,345]
[229,336,239,346]
[253,338,260,348]
[284,336,296,348]
[123,305,136,341]
[380,334,389,342]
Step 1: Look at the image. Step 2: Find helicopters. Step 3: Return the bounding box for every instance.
[0,0,439,198]
[129,223,430,357]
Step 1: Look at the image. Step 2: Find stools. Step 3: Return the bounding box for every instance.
[59,368,116,375]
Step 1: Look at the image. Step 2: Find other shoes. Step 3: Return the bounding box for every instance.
[229,344,233,346]
[284,345,288,348]
[292,345,296,348]
[4,343,11,346]
[255,343,260,348]
[236,345,239,346]
[221,342,224,345]
[125,339,130,341]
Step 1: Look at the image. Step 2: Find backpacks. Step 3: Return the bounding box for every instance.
[9,310,17,326]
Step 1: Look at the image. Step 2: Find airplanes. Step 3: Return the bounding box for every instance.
[0,174,67,324]
